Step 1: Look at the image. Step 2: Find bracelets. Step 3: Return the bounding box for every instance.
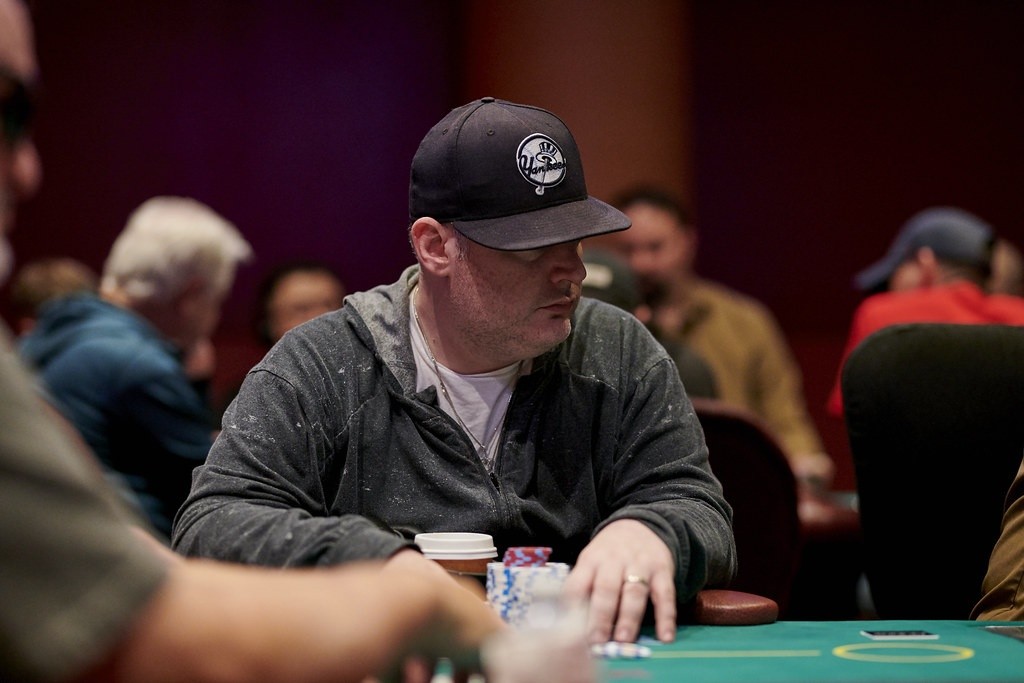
[623,577,649,587]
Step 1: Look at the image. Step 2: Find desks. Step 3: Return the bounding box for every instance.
[417,620,1024,683]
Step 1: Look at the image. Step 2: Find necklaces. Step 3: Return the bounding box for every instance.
[413,286,511,473]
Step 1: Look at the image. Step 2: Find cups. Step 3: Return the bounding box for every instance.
[415,533,497,574]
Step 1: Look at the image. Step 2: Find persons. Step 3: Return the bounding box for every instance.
[828,207,1024,624]
[171,98,735,644]
[16,258,93,340]
[0,0,507,683]
[22,197,251,541]
[256,258,346,350]
[579,188,835,617]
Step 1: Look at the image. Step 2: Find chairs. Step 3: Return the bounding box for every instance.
[842,322,1024,619]
[691,395,863,621]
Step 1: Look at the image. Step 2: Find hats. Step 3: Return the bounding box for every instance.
[860,206,992,288]
[578,255,634,313]
[410,97,633,252]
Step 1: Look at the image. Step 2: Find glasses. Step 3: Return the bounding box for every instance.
[0,69,43,146]
